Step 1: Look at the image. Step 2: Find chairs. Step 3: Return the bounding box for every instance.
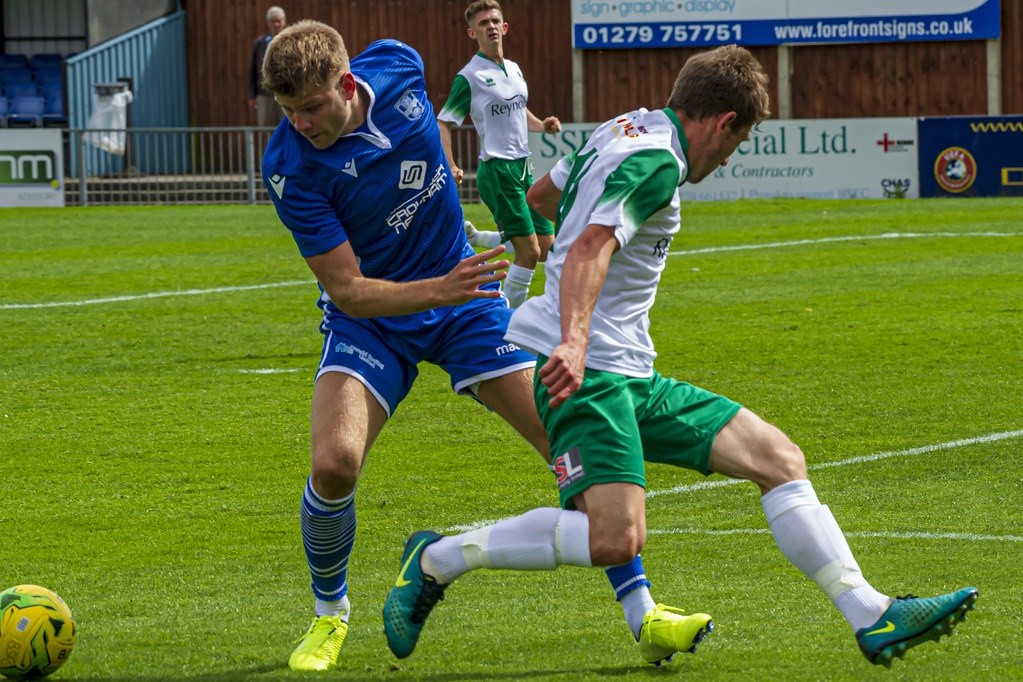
[0,49,84,129]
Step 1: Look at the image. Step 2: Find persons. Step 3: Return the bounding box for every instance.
[382,42,978,670]
[261,19,715,671]
[436,0,563,309]
[248,7,286,178]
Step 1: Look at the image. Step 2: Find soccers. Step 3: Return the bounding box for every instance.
[0,584,76,681]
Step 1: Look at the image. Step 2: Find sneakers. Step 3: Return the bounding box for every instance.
[633,603,714,665]
[381,531,449,658]
[289,610,348,670]
[856,586,978,667]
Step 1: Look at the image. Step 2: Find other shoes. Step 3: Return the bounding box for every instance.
[464,221,478,238]
